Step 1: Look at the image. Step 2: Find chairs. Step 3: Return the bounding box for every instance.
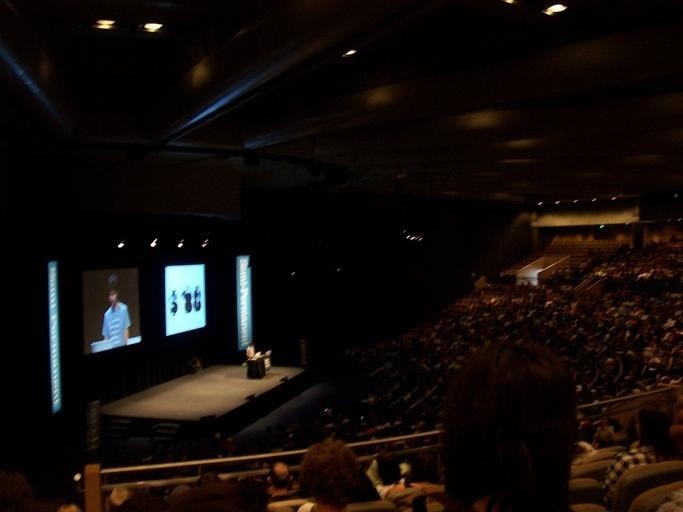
[267,445,683,511]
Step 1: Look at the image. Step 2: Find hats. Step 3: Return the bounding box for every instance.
[106,273,119,288]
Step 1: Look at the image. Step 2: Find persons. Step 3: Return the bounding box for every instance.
[2,234,683,511]
